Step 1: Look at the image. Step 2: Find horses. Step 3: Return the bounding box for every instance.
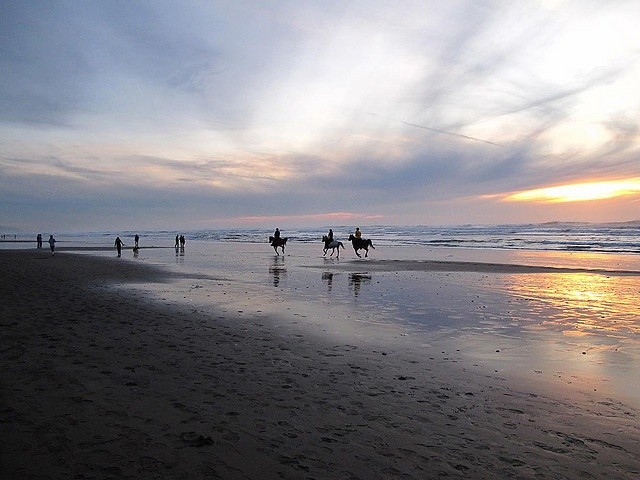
[269,235,290,255]
[321,234,346,259]
[348,233,376,259]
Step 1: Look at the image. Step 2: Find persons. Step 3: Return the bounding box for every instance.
[49,235,56,255]
[175,234,179,248]
[134,234,139,249]
[274,228,280,244]
[40,234,43,248]
[180,234,183,249]
[328,229,334,244]
[355,227,362,250]
[37,234,40,249]
[114,236,125,257]
[183,236,185,248]
